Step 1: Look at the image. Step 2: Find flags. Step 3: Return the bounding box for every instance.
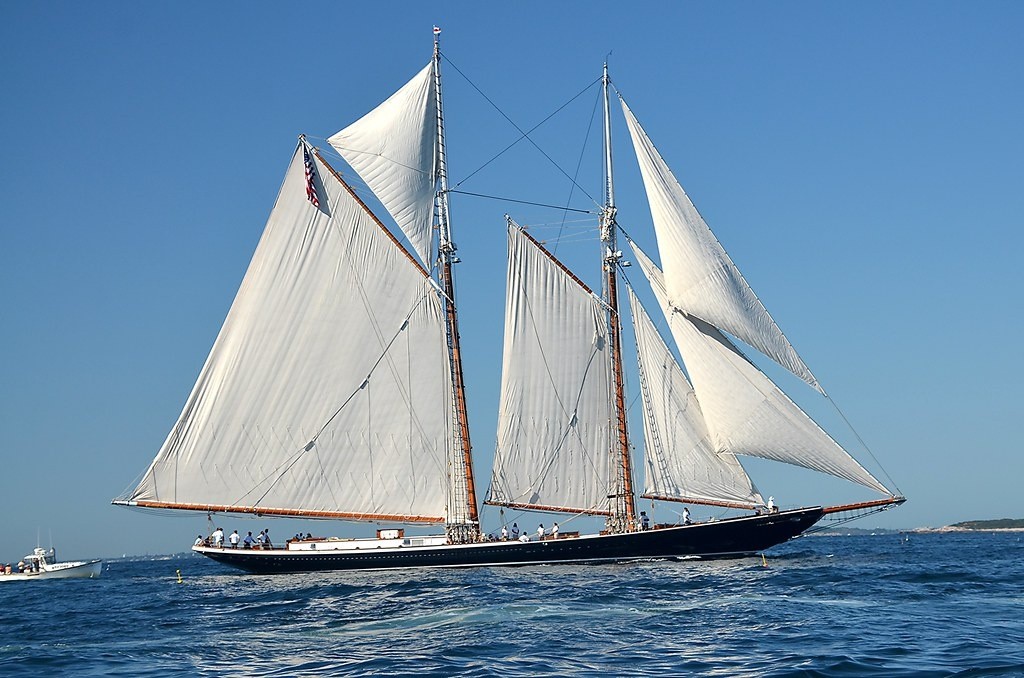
[304,144,320,207]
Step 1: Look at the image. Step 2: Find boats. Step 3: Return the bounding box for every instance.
[0,527,102,582]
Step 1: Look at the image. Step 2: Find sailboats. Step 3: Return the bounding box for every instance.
[110,26,906,576]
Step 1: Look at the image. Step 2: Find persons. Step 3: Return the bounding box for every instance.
[229,530,240,549]
[512,523,520,538]
[639,511,649,530]
[195,535,202,545]
[211,527,225,548]
[519,532,529,541]
[768,496,774,507]
[683,507,691,525]
[264,528,273,549]
[537,524,545,540]
[18,557,40,573]
[243,532,256,547]
[257,531,266,547]
[502,525,509,538]
[0,563,12,574]
[295,532,312,539]
[550,522,559,539]
